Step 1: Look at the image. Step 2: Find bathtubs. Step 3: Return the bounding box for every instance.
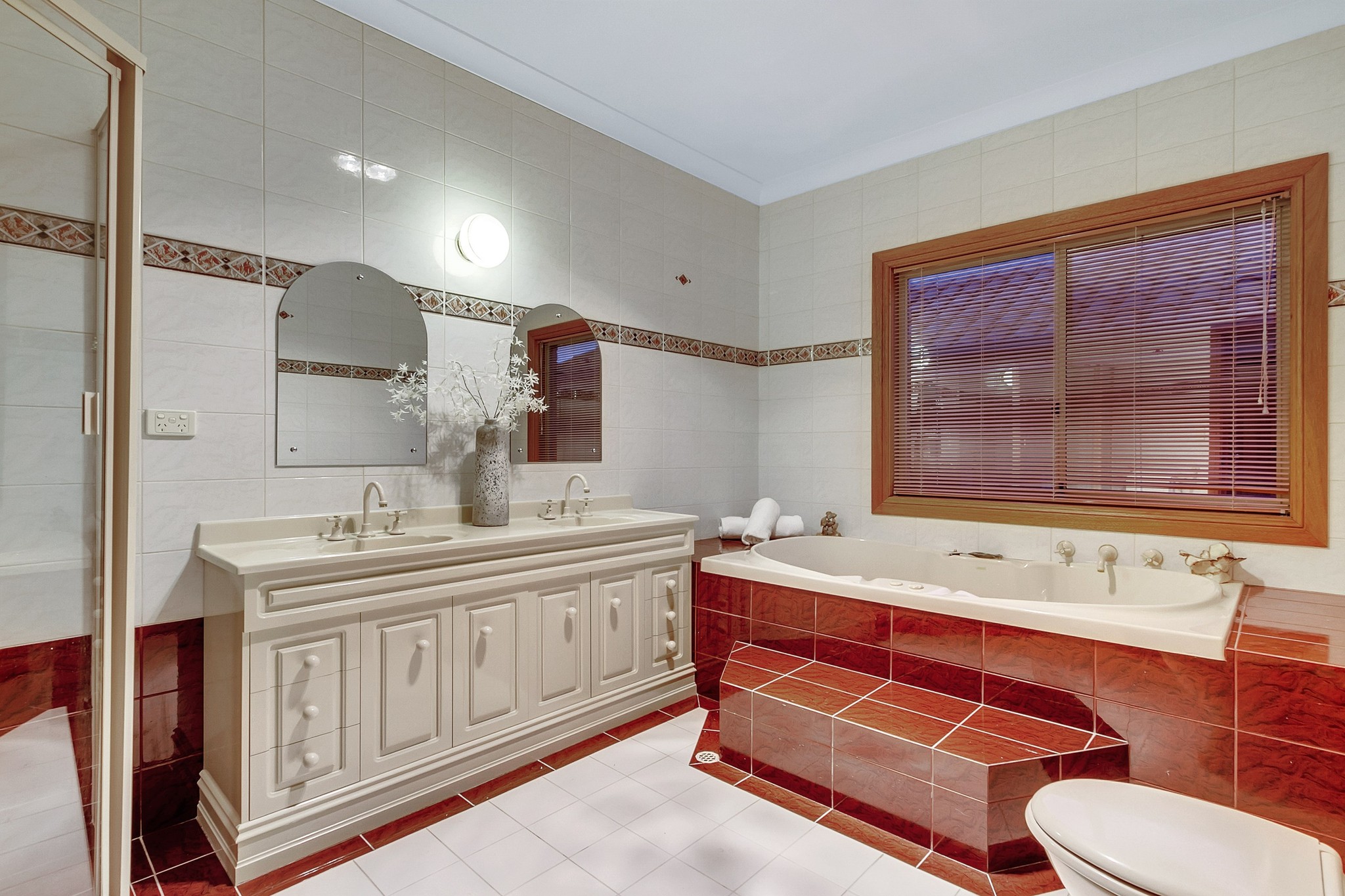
[700,535,1244,664]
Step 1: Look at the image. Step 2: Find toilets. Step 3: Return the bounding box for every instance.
[1023,778,1345,896]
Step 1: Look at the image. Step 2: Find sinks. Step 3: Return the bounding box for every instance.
[321,536,454,553]
[548,517,636,527]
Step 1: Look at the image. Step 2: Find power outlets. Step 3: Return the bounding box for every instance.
[145,408,196,437]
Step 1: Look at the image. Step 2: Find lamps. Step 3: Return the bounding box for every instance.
[456,212,510,269]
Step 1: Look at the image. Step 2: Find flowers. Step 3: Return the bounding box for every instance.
[382,334,550,433]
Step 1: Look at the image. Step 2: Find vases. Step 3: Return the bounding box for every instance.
[472,417,510,527]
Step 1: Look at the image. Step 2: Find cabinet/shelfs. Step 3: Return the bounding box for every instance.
[195,523,698,885]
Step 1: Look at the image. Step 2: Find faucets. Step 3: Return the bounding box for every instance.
[326,481,408,541]
[537,473,593,520]
[1096,545,1118,573]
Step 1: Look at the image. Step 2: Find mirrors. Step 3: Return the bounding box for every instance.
[275,262,429,466]
[510,303,603,464]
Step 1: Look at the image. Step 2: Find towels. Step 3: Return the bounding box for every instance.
[741,497,780,545]
[718,515,805,539]
